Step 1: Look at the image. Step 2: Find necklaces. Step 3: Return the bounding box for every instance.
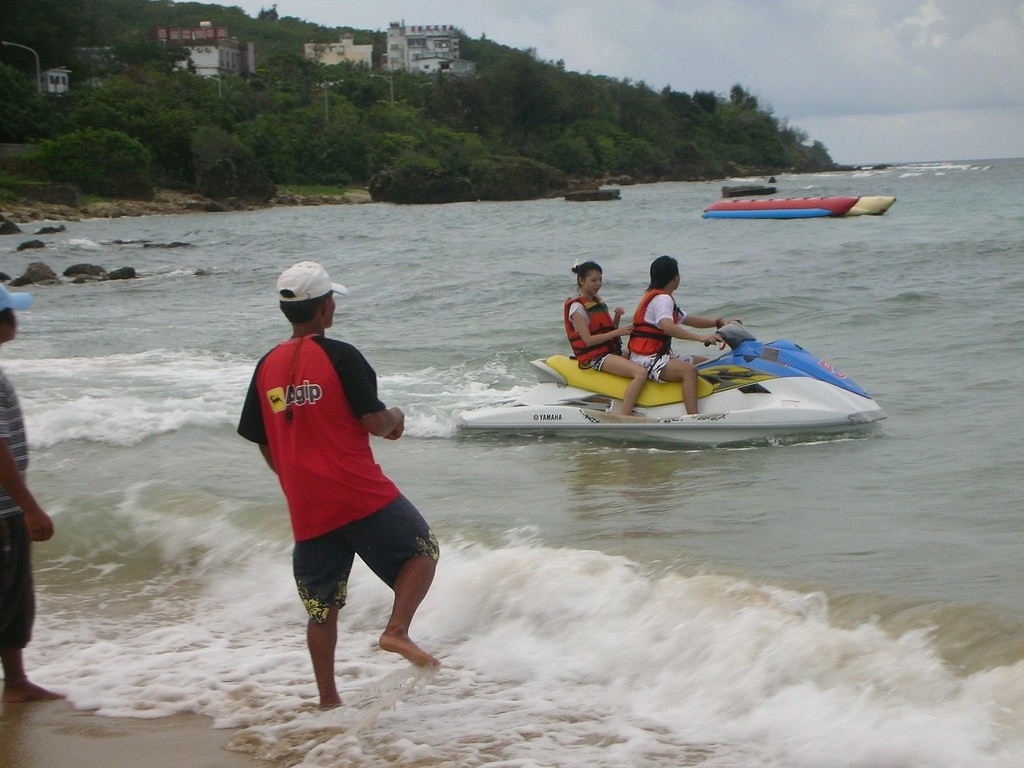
[285,334,307,422]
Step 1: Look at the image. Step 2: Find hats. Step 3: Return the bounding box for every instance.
[276,261,348,302]
[0,282,32,311]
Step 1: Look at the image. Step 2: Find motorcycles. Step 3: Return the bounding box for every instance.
[452,317,888,442]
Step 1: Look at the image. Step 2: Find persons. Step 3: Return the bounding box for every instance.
[237,260,440,708]
[564,261,648,415]
[628,255,742,415]
[0,282,65,702]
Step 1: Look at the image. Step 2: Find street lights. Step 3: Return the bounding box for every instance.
[371,73,396,104]
[1,40,41,94]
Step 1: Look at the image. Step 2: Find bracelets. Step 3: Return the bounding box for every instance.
[716,319,722,328]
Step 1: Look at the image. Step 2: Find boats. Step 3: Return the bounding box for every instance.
[699,194,901,220]
[719,177,779,195]
